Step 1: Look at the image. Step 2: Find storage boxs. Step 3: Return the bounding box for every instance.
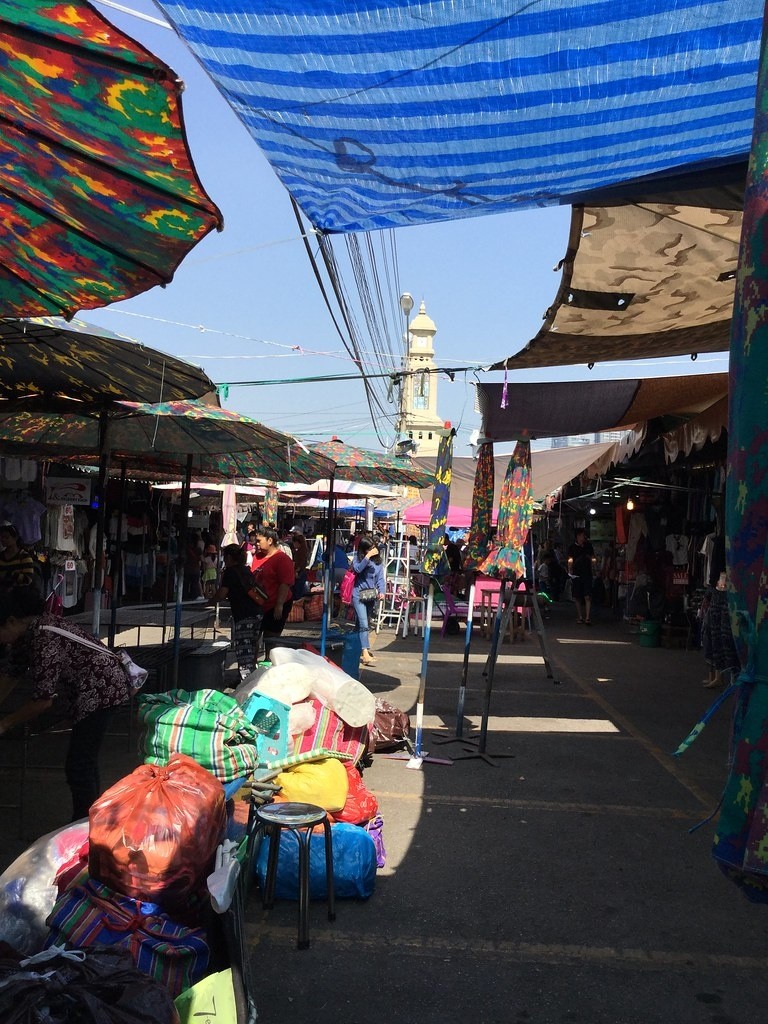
[639,622,690,647]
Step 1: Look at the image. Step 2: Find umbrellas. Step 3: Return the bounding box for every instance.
[0,394,336,639]
[304,434,437,535]
[0,317,221,407]
[0,0,221,324]
[415,422,535,755]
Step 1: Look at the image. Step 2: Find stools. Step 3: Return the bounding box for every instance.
[395,596,425,639]
[242,802,336,950]
[481,589,525,642]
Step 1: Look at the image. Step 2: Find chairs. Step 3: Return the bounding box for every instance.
[441,585,473,638]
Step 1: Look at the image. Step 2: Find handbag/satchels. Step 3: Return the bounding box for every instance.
[361,588,379,600]
[339,571,357,603]
[254,822,376,900]
[278,642,370,769]
[48,874,210,1000]
[227,759,346,813]
[117,649,147,690]
[91,753,227,912]
[361,819,387,868]
[131,691,257,784]
[373,701,407,752]
[249,586,271,607]
[0,941,180,1024]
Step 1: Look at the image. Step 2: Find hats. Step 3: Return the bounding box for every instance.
[205,545,218,553]
[289,525,301,532]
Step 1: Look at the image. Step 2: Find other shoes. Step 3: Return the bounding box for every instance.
[577,618,583,624]
[701,669,730,688]
[585,619,591,625]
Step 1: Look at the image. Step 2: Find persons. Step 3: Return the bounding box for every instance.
[530,531,616,624]
[704,570,740,688]
[0,520,34,594]
[442,534,462,578]
[206,543,264,682]
[249,522,296,662]
[287,530,451,601]
[0,584,131,822]
[351,536,386,667]
